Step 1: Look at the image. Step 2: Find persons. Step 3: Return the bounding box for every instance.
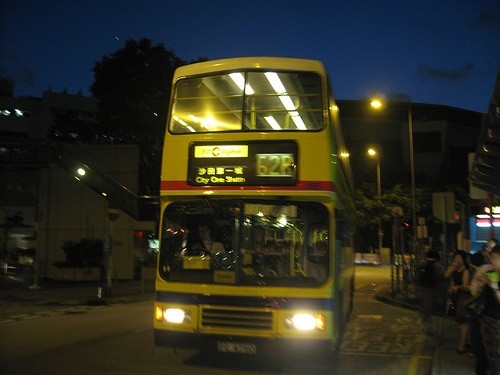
[443,240,500,375]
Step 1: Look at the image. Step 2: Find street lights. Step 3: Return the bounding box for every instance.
[367,148,383,266]
[369,93,418,285]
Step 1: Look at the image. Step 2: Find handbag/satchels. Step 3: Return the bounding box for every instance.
[445,300,456,316]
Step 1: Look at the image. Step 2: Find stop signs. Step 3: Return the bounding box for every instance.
[108,201,121,222]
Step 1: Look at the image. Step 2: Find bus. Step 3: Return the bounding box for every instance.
[153,54,356,362]
